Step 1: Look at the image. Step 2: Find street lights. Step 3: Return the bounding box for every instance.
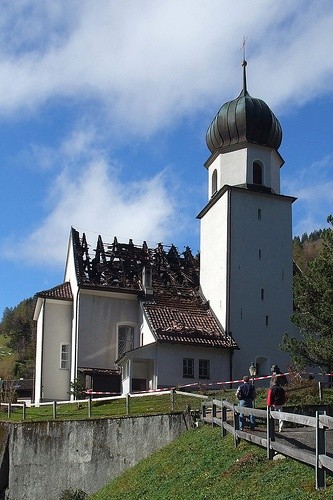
[248,362,257,409]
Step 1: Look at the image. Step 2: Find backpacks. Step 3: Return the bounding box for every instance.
[272,386,286,405]
[242,383,255,401]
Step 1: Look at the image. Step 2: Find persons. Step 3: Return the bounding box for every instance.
[236,376,256,431]
[267,375,285,433]
